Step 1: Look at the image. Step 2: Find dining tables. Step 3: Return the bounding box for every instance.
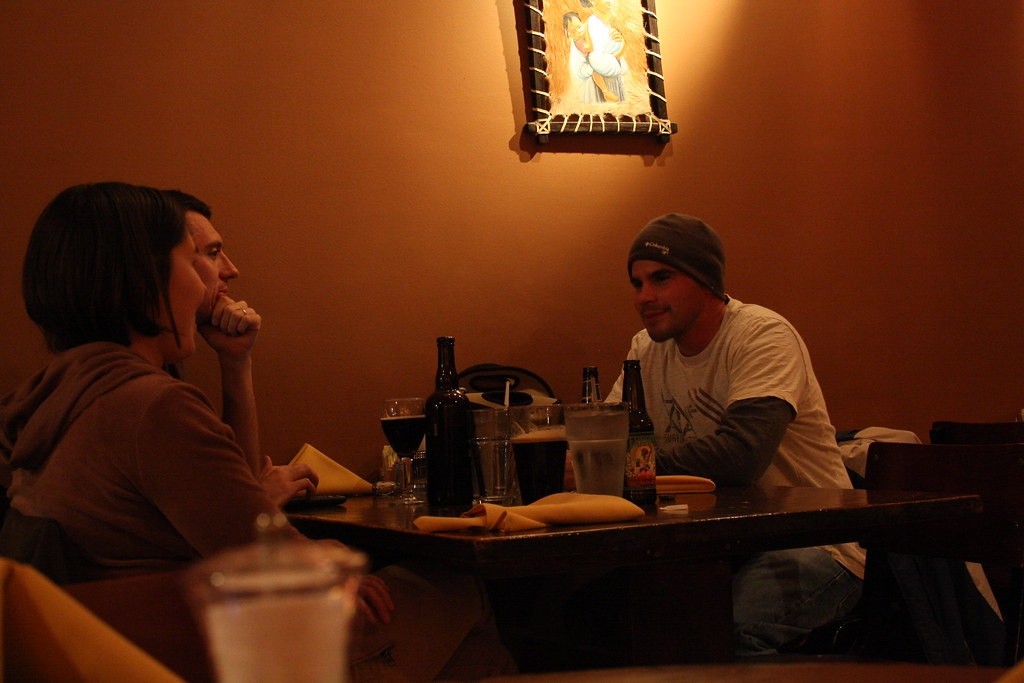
[275,479,979,664]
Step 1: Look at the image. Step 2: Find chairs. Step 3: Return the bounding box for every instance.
[807,421,1024,666]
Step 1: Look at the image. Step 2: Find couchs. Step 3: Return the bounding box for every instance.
[0,540,374,682]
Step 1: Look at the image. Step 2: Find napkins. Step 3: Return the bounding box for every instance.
[413,492,644,536]
[656,475,715,492]
[289,442,373,495]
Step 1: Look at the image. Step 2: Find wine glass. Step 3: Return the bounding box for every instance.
[379,397,430,502]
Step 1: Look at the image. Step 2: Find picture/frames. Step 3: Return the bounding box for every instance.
[516,0,678,145]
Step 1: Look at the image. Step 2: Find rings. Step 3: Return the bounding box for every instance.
[243,309,247,315]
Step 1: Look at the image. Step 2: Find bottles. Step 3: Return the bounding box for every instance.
[580,365,602,404]
[621,359,658,505]
[425,335,474,511]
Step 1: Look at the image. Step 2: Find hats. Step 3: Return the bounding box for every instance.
[626,212,725,300]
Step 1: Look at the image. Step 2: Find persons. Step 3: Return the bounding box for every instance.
[156,190,318,507]
[563,214,869,658]
[0,184,520,683]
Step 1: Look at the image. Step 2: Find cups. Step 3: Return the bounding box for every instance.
[507,405,570,506]
[563,401,630,498]
[183,538,367,683]
[473,405,527,504]
